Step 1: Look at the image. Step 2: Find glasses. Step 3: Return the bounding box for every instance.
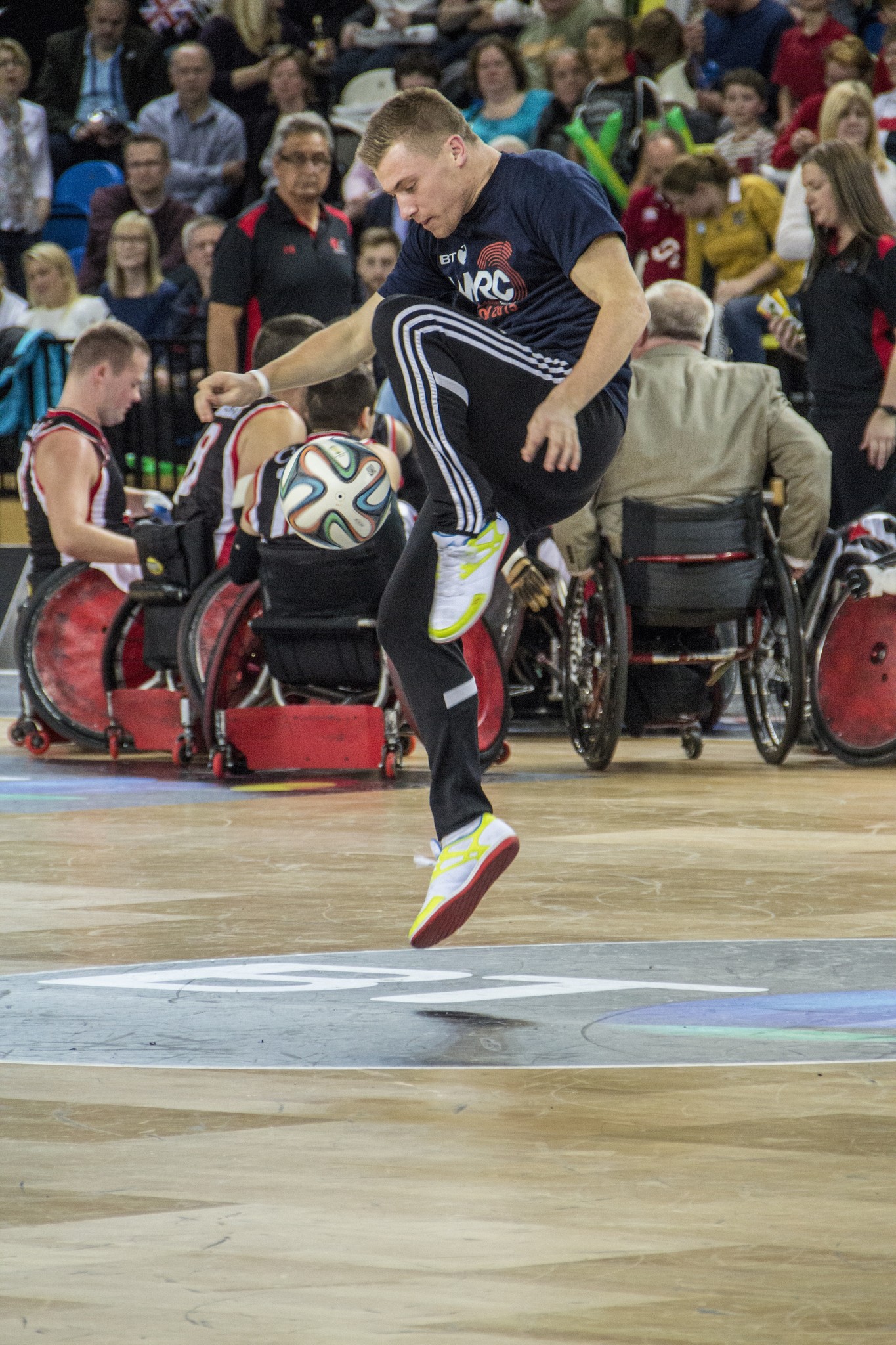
[283,151,333,167]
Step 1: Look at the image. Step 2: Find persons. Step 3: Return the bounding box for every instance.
[0,0,896,627]
[194,86,652,951]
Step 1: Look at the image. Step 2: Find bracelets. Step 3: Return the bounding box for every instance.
[876,403,895,417]
[244,370,270,400]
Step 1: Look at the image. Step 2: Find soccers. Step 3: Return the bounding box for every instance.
[279,435,392,551]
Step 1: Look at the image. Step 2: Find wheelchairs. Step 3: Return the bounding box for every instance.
[782,508,895,767]
[9,495,563,785]
[562,475,808,773]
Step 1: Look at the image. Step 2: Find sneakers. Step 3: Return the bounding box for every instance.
[426,510,511,645]
[407,812,521,949]
